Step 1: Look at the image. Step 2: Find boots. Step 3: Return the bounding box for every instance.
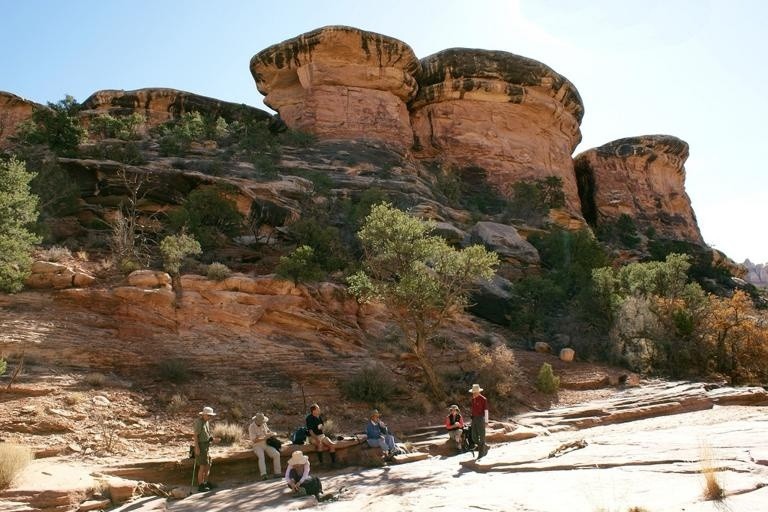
[316,492,334,502]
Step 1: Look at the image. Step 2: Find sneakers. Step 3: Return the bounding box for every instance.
[198,483,211,491]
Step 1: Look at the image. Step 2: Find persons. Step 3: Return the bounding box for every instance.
[284,450,333,501]
[192,406,221,492]
[468,383,491,459]
[366,408,398,460]
[445,404,465,452]
[305,404,342,468]
[248,411,286,480]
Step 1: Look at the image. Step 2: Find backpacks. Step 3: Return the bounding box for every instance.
[462,427,475,451]
[291,427,307,445]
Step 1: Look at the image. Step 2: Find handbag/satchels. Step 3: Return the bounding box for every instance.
[189,445,196,458]
[267,437,281,448]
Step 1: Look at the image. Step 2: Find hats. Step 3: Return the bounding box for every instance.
[449,405,459,411]
[287,450,309,464]
[369,409,382,418]
[252,413,268,422]
[469,383,483,393]
[199,407,216,416]
